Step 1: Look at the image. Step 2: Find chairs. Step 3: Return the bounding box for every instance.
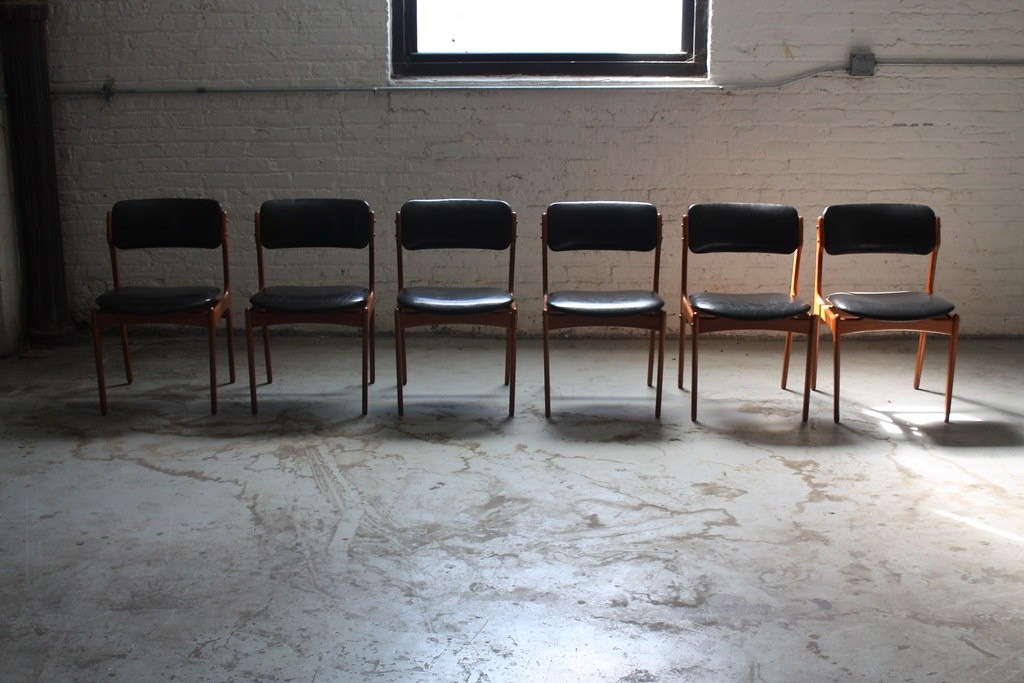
[678,202,816,423]
[244,199,377,416]
[541,201,668,419]
[394,198,519,417]
[810,202,961,424]
[90,197,236,416]
